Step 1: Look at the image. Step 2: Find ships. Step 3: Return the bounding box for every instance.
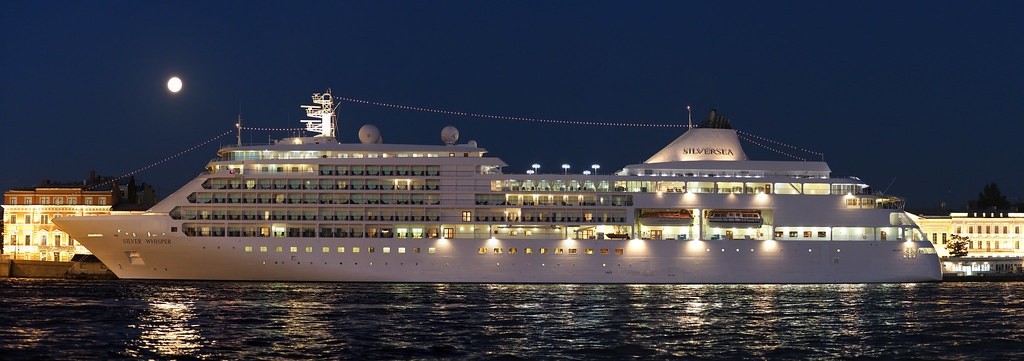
[50,89,944,284]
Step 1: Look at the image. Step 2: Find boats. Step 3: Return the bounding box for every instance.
[707,211,762,227]
[638,210,693,226]
